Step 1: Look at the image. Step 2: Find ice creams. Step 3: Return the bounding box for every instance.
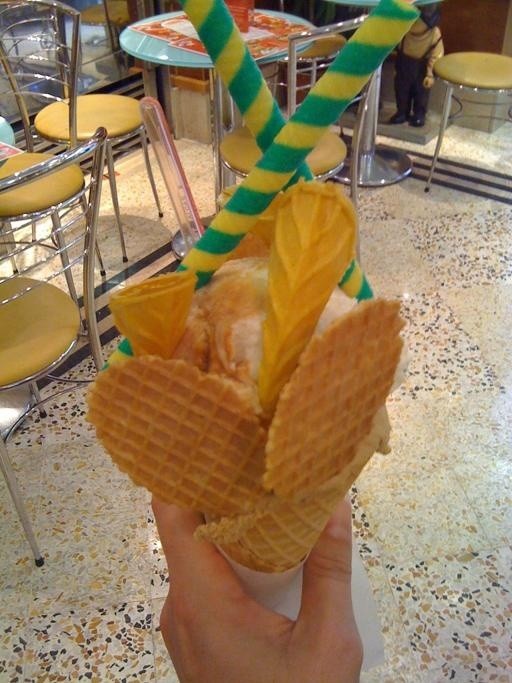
[87,182,405,572]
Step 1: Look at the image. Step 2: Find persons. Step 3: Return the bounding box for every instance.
[390,3,445,127]
[150,490,368,683]
